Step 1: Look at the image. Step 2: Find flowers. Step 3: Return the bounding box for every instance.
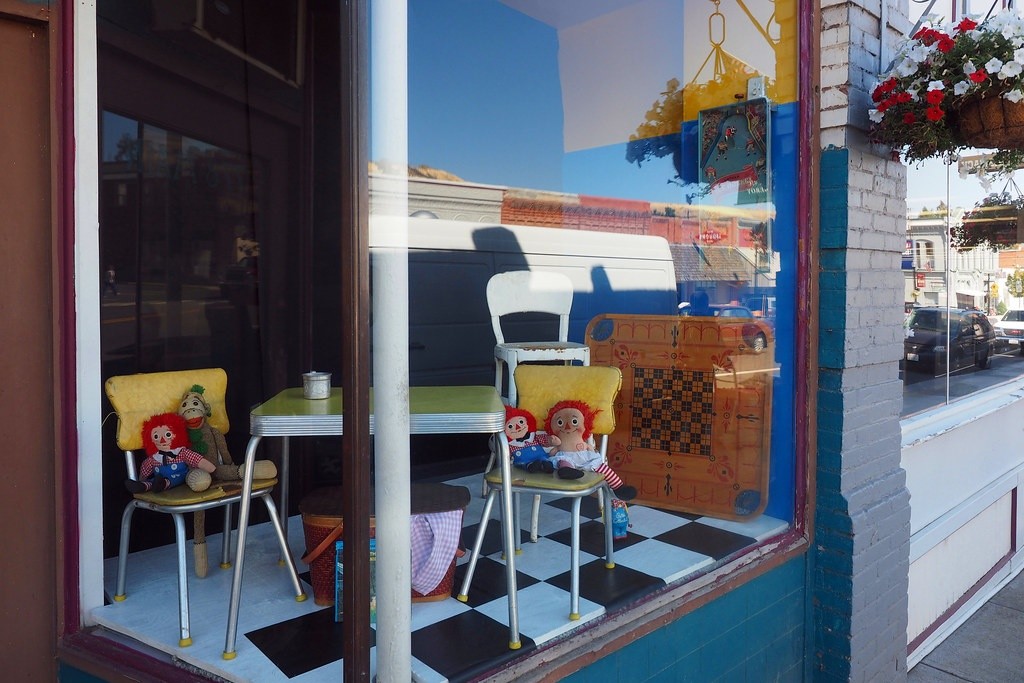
[946,192,1024,254]
[627,64,770,206]
[870,7,1024,179]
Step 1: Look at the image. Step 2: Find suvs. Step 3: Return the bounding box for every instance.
[899,306,1006,370]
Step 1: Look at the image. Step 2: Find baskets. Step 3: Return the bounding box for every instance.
[299,479,467,606]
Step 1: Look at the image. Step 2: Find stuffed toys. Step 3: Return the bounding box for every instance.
[544,401,637,501]
[178,386,277,491]
[126,412,216,493]
[503,404,553,473]
[602,499,632,540]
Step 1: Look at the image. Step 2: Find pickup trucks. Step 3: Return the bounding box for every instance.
[743,297,776,318]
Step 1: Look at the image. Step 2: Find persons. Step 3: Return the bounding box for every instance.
[702,103,767,182]
[103,264,120,298]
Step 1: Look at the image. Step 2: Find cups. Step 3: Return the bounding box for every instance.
[302,371,332,399]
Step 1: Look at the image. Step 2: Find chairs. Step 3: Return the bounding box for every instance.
[458,365,623,621]
[482,268,591,543]
[106,368,307,648]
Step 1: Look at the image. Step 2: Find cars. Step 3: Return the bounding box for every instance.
[993,309,1024,354]
[677,304,774,356]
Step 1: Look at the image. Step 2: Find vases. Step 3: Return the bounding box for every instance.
[672,142,700,183]
[959,90,1024,150]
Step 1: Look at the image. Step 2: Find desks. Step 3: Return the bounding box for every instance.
[222,386,522,661]
